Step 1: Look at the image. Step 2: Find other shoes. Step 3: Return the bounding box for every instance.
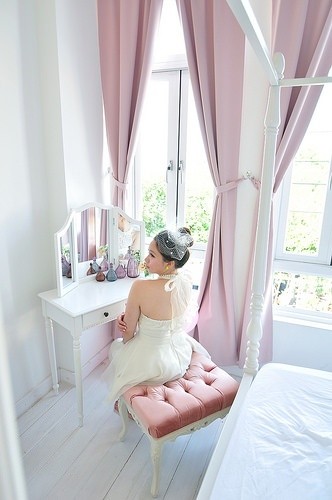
[114,400,134,421]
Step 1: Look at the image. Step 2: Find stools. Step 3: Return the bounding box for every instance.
[118,351,239,497]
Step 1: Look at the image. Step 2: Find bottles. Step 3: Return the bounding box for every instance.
[95,266,105,282]
[87,263,96,275]
[66,263,72,278]
[100,256,109,271]
[61,258,68,276]
[92,259,100,272]
[115,260,126,279]
[106,263,118,282]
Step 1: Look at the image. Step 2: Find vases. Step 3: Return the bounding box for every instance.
[145,273,150,277]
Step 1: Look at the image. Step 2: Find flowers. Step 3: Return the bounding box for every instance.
[37,202,156,426]
[138,262,149,273]
[98,245,108,254]
[63,247,71,260]
[133,249,140,261]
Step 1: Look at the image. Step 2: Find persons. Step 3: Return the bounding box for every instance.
[101,227,199,421]
[118,213,140,259]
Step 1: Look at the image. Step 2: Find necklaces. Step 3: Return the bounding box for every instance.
[159,274,177,280]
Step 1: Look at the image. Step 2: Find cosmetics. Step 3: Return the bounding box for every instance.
[118,246,140,259]
[87,254,109,277]
[95,253,140,282]
[61,256,72,280]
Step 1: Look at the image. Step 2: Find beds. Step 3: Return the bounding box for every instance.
[196,0,332,500]
